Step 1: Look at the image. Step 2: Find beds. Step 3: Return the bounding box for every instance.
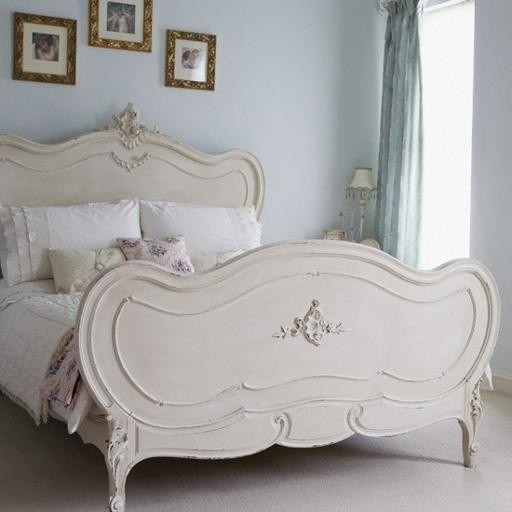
[0,101,502,511]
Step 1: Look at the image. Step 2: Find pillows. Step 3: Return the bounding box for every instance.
[0,197,264,296]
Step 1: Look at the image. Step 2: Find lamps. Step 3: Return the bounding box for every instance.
[345,166,380,242]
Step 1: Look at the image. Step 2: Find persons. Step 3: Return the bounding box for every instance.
[182,48,202,69]
[113,9,130,32]
[35,35,58,62]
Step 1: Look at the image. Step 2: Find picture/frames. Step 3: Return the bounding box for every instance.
[11,0,217,92]
